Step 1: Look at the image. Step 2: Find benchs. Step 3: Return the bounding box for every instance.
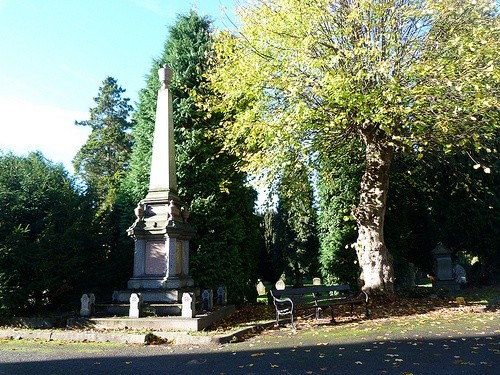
[270,283,370,328]
